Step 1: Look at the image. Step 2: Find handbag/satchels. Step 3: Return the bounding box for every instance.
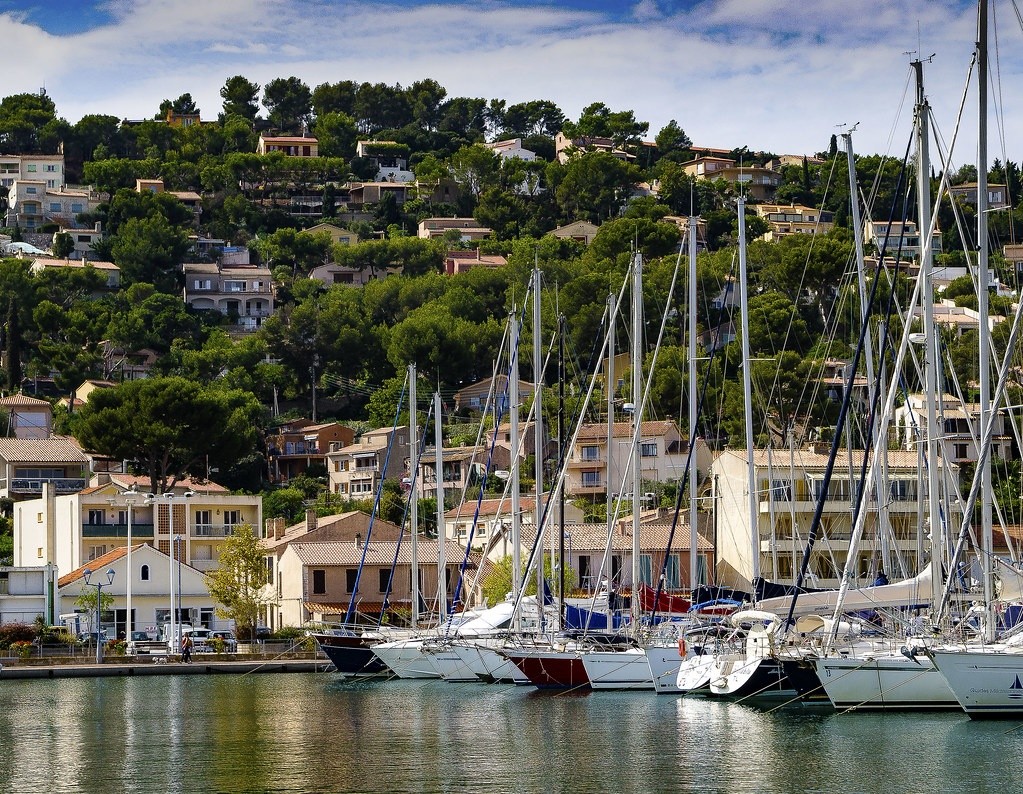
[186,638,192,647]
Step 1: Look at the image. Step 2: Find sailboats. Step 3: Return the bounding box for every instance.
[309,0,1023,720]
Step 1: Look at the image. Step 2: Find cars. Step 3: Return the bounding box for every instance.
[126,622,270,655]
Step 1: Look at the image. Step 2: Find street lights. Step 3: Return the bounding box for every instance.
[82,568,115,664]
[174,534,183,649]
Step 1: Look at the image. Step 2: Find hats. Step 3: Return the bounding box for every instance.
[972,600,976,605]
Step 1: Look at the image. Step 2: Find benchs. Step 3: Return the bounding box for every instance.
[132,641,172,653]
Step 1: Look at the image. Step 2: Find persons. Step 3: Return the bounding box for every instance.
[132,482,140,489]
[180,632,192,664]
